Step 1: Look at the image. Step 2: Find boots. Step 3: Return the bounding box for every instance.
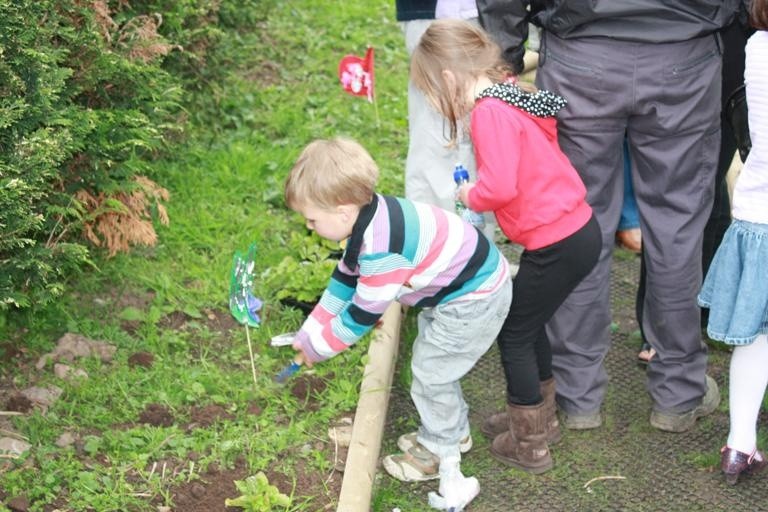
[481,382,560,473]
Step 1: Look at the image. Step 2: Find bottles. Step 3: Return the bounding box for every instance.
[451,163,486,233]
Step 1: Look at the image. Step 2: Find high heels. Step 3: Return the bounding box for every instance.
[721,445,768,484]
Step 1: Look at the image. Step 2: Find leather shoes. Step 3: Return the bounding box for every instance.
[616,228,643,251]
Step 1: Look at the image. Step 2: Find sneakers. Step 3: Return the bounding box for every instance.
[383,431,474,481]
[651,374,720,431]
[556,397,604,429]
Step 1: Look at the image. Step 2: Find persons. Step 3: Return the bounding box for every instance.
[698,0,768,485]
[396,0,520,278]
[285,137,513,483]
[635,12,752,364]
[477,1,739,432]
[409,20,603,474]
